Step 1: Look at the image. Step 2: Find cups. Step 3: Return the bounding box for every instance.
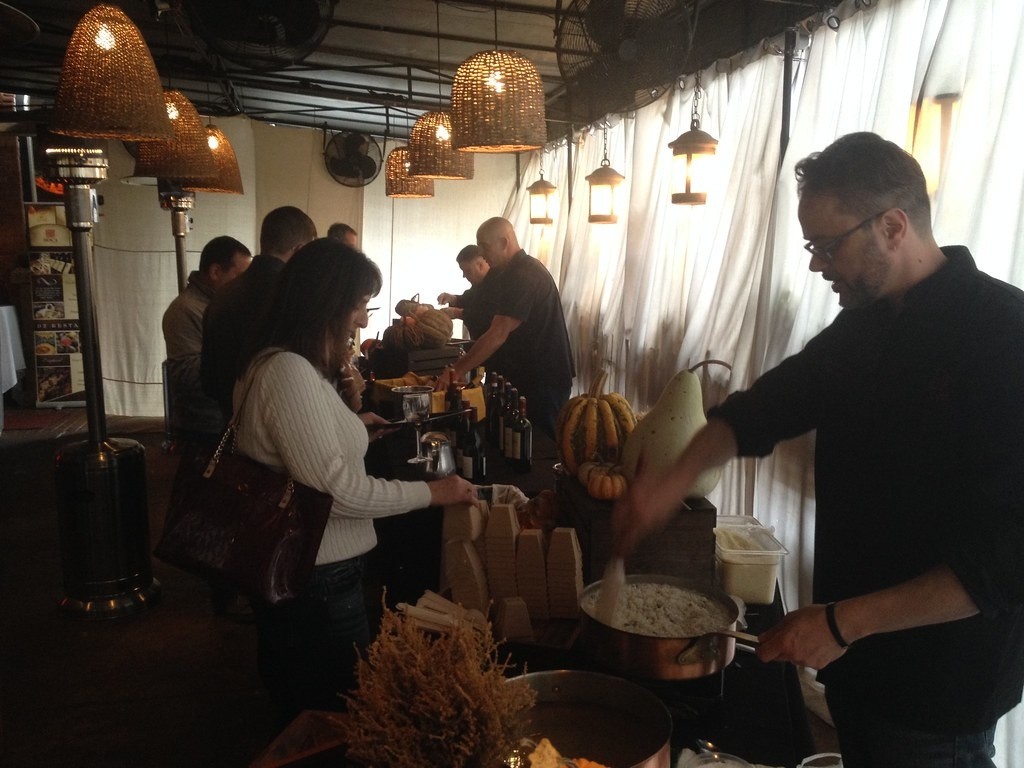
[426,441,456,480]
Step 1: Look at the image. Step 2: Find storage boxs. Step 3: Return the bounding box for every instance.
[713,515,790,605]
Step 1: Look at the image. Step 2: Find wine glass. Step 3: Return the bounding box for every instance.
[403,394,433,463]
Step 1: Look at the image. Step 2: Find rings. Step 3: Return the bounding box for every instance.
[464,487,474,496]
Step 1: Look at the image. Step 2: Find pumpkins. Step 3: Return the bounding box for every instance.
[556,368,638,500]
[360,293,453,355]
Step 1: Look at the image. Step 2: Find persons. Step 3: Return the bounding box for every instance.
[162,206,366,506]
[231,237,480,716]
[434,218,577,440]
[607,132,1024,768]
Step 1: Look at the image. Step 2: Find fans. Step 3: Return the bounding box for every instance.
[322,108,390,188]
[552,1,700,113]
[181,0,339,72]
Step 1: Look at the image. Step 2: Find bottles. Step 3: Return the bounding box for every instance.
[488,372,505,453]
[512,397,532,474]
[445,370,489,483]
[504,382,518,466]
[367,369,375,398]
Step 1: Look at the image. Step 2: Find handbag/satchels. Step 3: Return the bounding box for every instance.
[154,349,333,602]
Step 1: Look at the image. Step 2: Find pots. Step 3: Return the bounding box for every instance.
[578,574,760,680]
[505,670,672,768]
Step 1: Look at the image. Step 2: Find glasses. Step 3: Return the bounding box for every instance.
[804,210,909,265]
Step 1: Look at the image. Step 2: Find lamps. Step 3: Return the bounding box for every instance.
[450,1,547,154]
[585,122,625,224]
[48,0,174,140]
[386,147,435,200]
[668,69,717,204]
[182,65,243,194]
[407,0,475,179]
[527,154,555,223]
[133,12,218,177]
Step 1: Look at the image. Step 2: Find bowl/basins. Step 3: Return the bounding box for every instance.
[391,386,434,419]
[685,752,753,768]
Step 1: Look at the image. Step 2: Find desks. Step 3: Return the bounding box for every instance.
[385,416,564,615]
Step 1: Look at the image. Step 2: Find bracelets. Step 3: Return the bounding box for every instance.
[824,602,850,649]
